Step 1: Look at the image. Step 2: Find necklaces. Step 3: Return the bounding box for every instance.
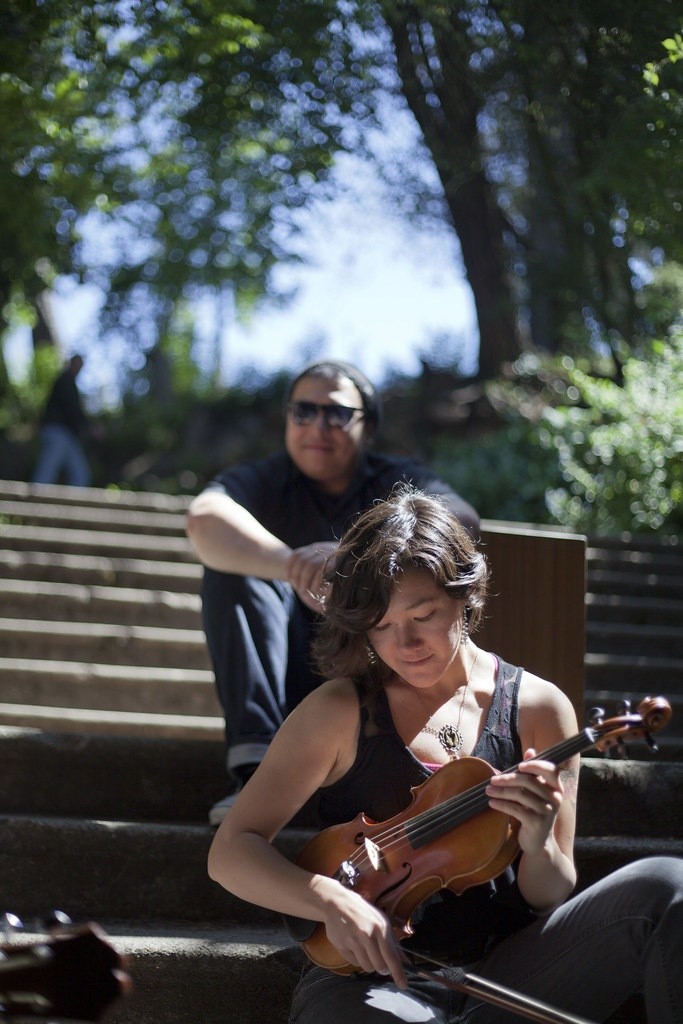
[405,652,478,754]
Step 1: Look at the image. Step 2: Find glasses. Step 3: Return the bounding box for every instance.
[287,400,367,427]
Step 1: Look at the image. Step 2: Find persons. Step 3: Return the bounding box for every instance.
[186,363,481,828]
[206,480,683,1023]
[31,355,97,487]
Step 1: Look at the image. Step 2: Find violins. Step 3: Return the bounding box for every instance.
[282,694,677,979]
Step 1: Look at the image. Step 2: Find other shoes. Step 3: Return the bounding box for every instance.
[209,782,245,825]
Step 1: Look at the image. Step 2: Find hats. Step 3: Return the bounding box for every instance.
[291,360,380,426]
